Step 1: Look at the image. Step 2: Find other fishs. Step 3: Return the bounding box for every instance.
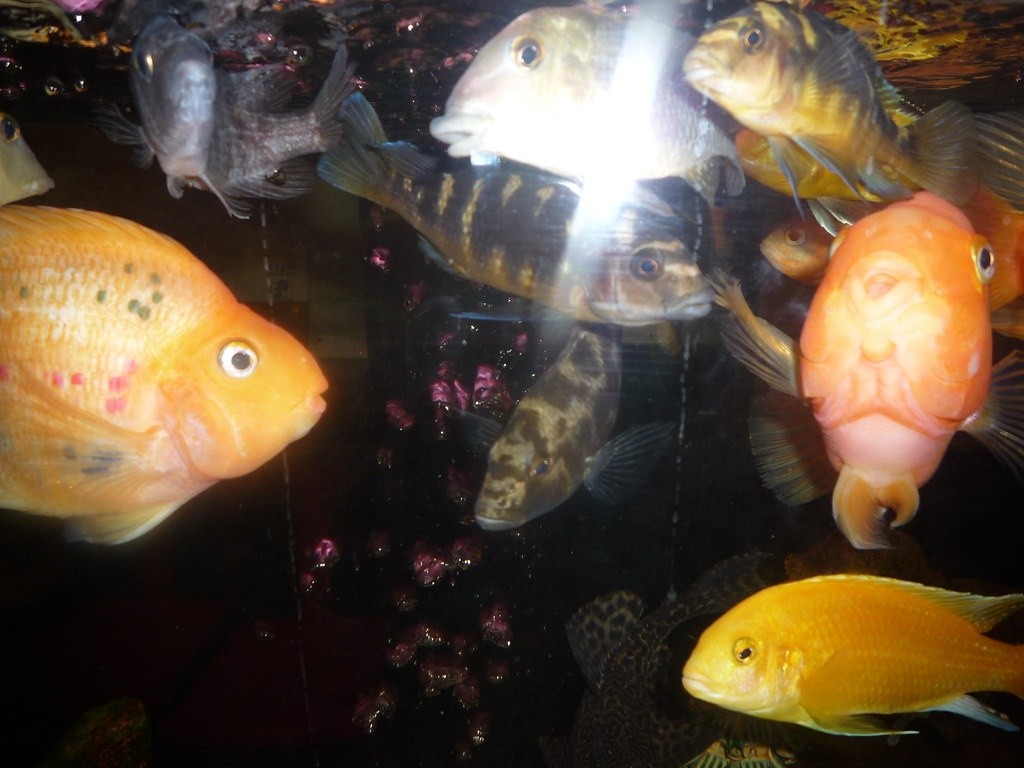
[5,1,1024,768]
